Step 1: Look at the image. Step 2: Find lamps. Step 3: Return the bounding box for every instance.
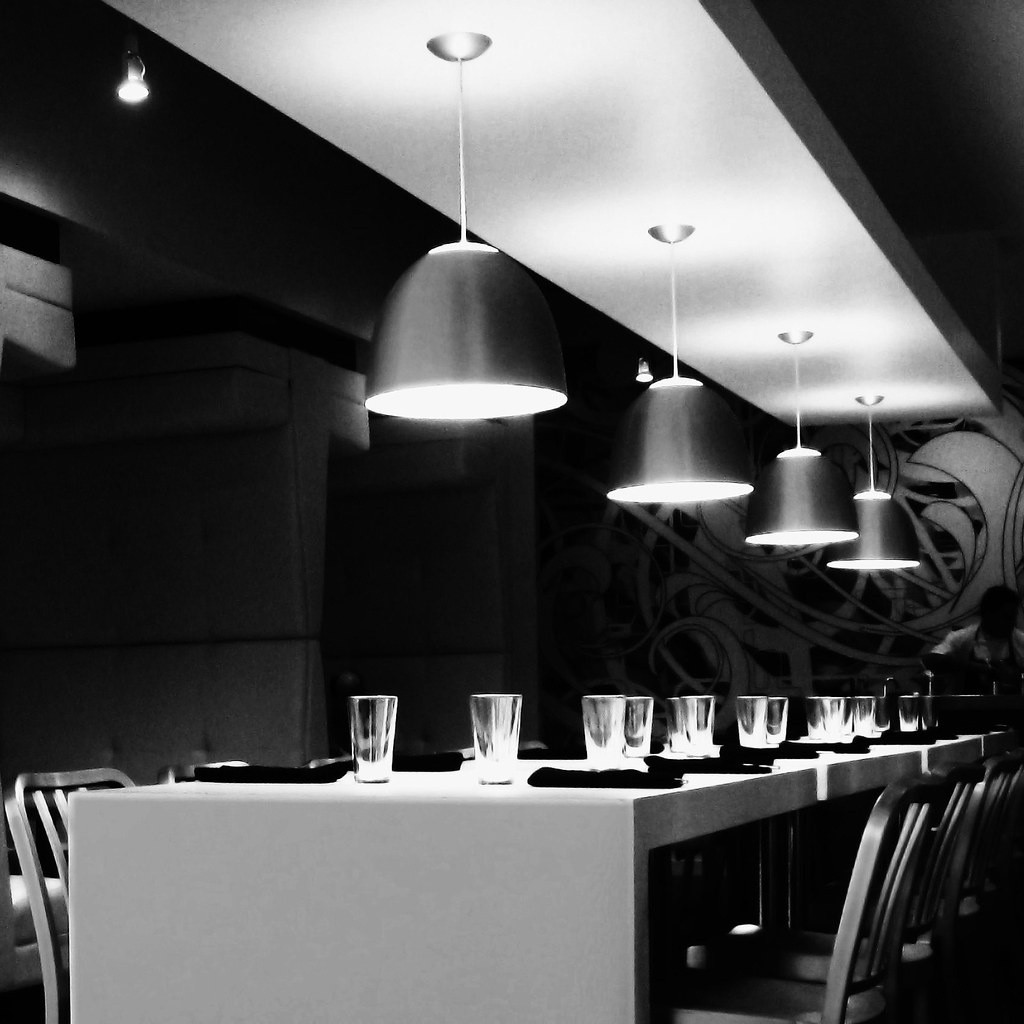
[115,51,150,102]
[744,331,858,547]
[827,395,920,569]
[367,31,569,420]
[606,221,755,504]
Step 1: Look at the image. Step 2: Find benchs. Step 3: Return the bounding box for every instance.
[0,799,69,995]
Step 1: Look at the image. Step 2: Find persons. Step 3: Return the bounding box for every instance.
[931,584,1024,668]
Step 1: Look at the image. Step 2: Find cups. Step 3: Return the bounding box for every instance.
[681,697,715,756]
[807,697,890,740]
[766,697,789,743]
[919,696,939,730]
[470,694,522,785]
[583,695,626,773]
[899,696,919,732]
[667,698,684,753]
[349,697,398,782]
[736,696,766,748]
[622,697,653,758]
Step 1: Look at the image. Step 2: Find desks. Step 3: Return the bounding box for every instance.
[67,729,1010,1024]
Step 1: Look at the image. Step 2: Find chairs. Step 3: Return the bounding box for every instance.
[655,748,1024,1024]
[4,760,251,1023]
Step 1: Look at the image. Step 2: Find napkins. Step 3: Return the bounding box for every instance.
[641,755,773,775]
[850,736,937,747]
[526,765,683,790]
[192,761,348,785]
[926,725,996,734]
[516,748,587,762]
[778,740,872,755]
[343,751,462,773]
[717,743,821,760]
[881,730,956,741]
